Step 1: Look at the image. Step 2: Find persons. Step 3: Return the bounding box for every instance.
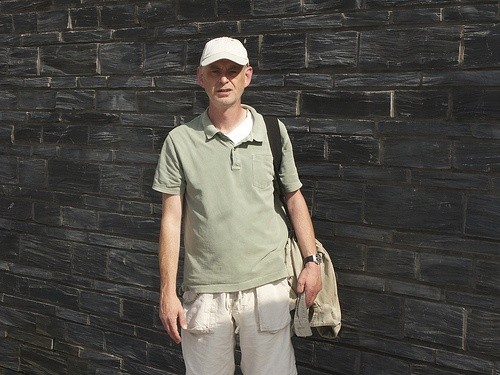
[151,36,324,375]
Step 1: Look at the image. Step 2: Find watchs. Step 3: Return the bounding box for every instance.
[303,253,322,265]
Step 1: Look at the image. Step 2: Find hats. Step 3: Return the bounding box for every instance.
[199,37,250,67]
